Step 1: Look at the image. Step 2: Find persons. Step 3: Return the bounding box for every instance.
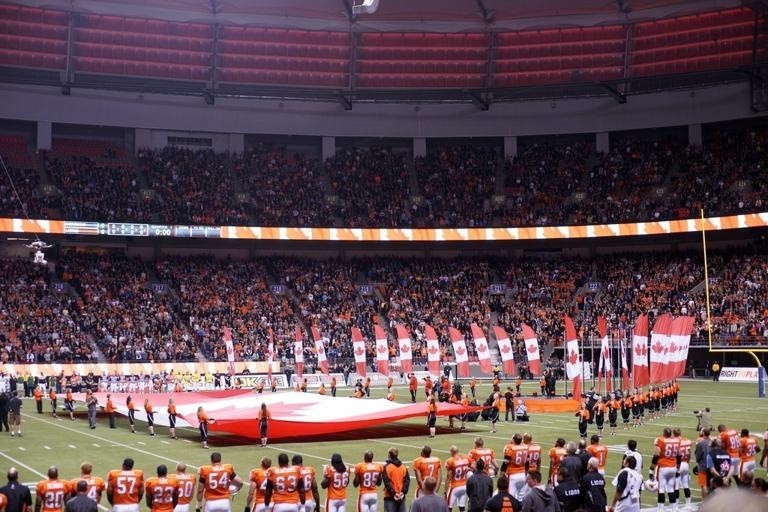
[0,345,456,386]
[1,370,767,512]
[493,359,564,379]
[688,359,720,382]
[589,358,596,374]
[443,363,452,376]
[0,137,767,347]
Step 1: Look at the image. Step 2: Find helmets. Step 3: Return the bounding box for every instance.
[487,463,496,479]
[645,479,659,493]
[228,481,240,495]
